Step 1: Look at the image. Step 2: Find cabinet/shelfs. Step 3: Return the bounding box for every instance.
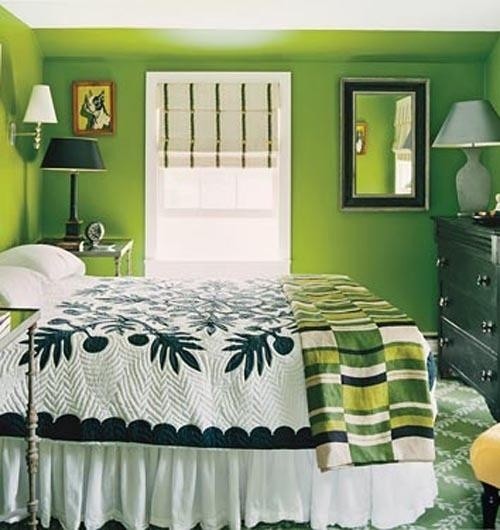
[429,216,500,422]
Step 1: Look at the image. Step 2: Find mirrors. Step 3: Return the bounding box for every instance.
[338,75,431,212]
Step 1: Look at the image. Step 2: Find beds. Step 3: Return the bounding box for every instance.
[0,270,437,530]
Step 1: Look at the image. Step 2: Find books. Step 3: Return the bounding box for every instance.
[53,241,84,249]
[0,310,11,338]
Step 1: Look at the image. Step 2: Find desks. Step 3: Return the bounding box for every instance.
[33,233,134,279]
[0,303,49,530]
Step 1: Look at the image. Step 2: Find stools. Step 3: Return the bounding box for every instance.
[467,421,500,528]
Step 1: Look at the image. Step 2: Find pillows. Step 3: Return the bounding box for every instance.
[0,265,77,307]
[0,240,83,280]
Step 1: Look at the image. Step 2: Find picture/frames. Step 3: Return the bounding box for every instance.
[354,121,367,154]
[71,78,117,139]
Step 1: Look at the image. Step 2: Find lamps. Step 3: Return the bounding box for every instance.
[37,133,107,241]
[7,78,62,150]
[431,95,500,214]
